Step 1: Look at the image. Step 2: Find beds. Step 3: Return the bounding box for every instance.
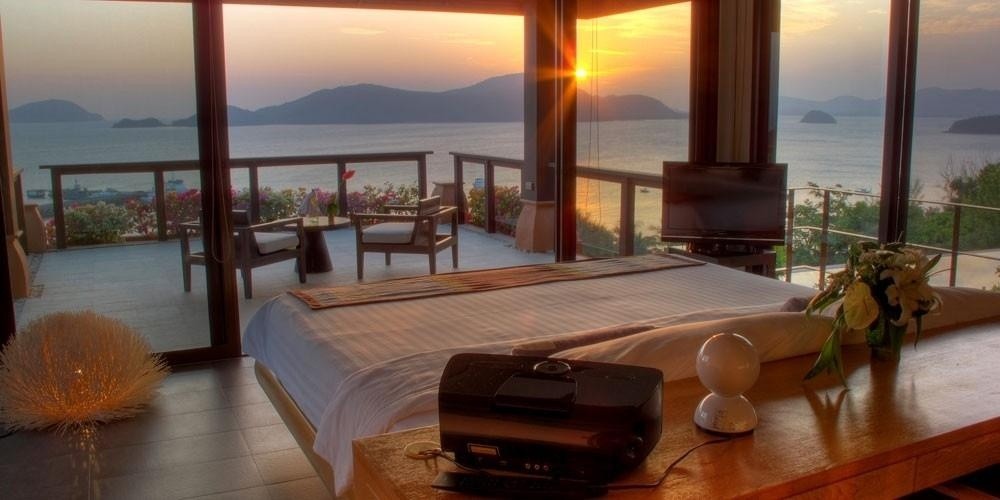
[240,247,999,500]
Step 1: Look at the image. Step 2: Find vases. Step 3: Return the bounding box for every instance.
[864,310,907,365]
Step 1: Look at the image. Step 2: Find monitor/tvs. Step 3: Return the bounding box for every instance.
[662,161,788,254]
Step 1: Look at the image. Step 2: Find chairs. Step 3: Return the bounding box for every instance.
[177,208,306,300]
[353,195,460,281]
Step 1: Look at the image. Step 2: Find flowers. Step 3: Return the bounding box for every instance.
[801,228,955,392]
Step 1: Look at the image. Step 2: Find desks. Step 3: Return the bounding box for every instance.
[665,245,778,278]
[272,215,351,274]
[350,310,999,500]
